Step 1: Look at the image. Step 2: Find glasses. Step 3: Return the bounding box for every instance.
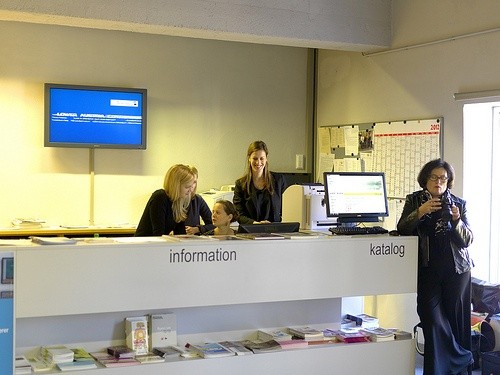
[429,174,449,182]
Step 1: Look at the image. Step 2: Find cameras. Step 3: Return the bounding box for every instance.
[432,202,453,222]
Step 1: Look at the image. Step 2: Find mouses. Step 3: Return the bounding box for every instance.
[389,230,399,236]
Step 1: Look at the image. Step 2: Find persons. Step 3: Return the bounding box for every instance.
[134,164,213,237]
[396,159,475,375]
[202,200,240,234]
[232,140,286,225]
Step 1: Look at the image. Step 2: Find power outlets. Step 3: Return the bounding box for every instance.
[295,154,304,169]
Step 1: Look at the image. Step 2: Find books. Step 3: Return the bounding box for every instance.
[235,232,320,240]
[288,314,412,345]
[15,314,308,375]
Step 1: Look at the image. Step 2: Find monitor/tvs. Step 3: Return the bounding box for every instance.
[323,172,389,226]
[44,82,147,150]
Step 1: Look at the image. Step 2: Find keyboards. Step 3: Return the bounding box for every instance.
[328,226,388,235]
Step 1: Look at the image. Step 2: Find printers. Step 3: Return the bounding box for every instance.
[281,183,339,230]
